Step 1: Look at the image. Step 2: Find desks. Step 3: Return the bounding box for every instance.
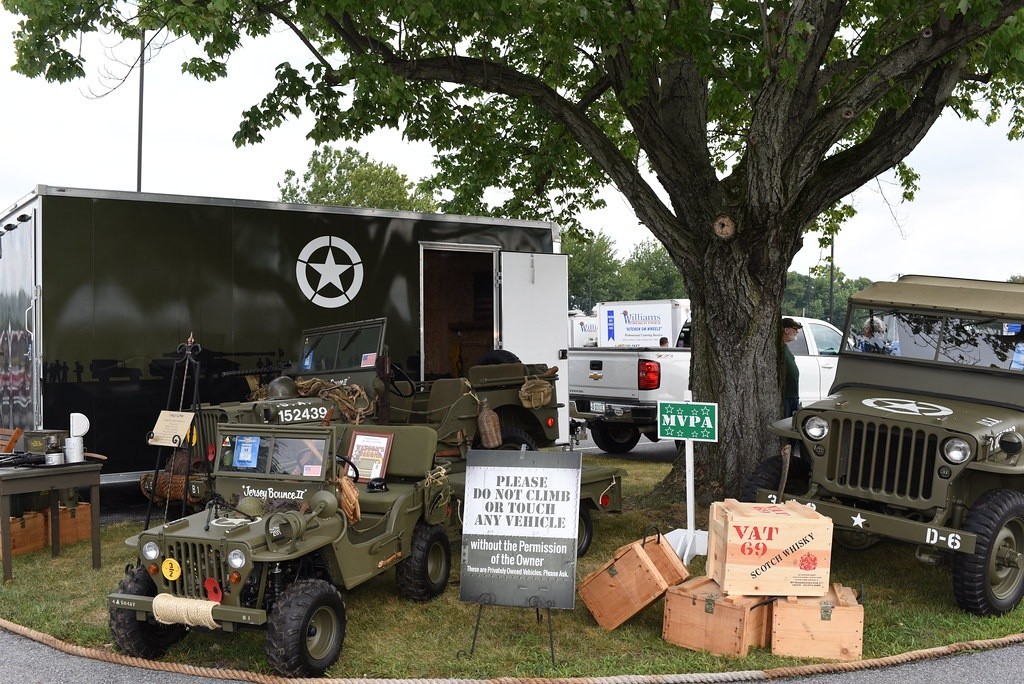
[0,463,104,585]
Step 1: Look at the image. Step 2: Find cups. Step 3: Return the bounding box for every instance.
[65,437,84,464]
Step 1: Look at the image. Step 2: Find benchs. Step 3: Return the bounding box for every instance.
[289,423,438,514]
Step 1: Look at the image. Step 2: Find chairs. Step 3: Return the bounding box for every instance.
[469,364,529,390]
[427,378,469,421]
[389,379,415,425]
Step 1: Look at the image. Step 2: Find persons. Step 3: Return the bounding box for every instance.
[859,317,888,353]
[778,318,804,420]
[660,337,668,347]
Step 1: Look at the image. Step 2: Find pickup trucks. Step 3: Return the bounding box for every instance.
[567,316,857,454]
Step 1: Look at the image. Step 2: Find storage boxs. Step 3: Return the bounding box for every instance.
[662,575,772,659]
[0,512,46,560]
[770,583,864,662]
[705,498,833,596]
[45,502,92,546]
[576,532,690,633]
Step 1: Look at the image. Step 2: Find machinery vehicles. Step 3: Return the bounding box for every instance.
[147,345,277,377]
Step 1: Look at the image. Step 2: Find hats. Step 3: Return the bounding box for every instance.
[781,318,803,329]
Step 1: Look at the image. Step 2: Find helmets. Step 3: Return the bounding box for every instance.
[264,376,300,399]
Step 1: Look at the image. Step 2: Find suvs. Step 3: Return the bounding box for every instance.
[138,317,564,522]
[89,359,143,383]
[107,421,453,678]
[756,274,1024,618]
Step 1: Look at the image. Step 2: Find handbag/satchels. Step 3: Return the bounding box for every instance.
[519,375,553,410]
